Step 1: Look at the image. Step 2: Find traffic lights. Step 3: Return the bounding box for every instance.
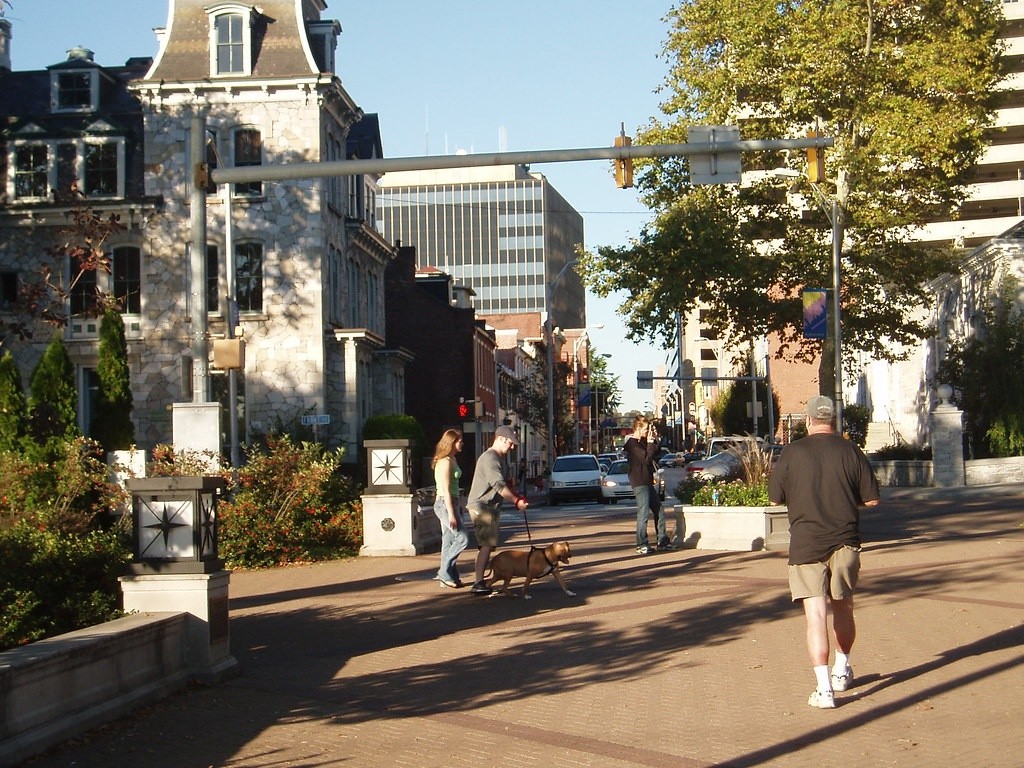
[459,404,468,417]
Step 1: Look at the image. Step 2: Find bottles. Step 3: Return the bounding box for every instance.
[712,489,718,507]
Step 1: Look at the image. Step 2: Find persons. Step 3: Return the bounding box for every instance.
[768,396,880,708]
[431,429,469,588]
[623,414,681,553]
[465,425,529,595]
[518,457,528,496]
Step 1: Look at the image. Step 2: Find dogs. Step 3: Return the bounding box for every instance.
[483,540,579,600]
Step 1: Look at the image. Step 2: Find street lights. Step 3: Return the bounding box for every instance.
[774,167,842,433]
[588,353,613,456]
[573,322,605,455]
[204,129,241,472]
[547,258,580,472]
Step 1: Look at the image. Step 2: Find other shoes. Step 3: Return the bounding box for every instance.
[437,577,464,588]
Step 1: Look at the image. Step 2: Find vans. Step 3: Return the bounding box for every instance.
[700,436,764,460]
[544,455,605,507]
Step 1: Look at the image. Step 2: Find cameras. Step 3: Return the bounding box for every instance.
[650,425,656,431]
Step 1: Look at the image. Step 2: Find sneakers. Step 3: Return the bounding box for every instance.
[808,690,835,708]
[657,543,681,551]
[636,546,656,554]
[470,580,493,595]
[831,663,853,692]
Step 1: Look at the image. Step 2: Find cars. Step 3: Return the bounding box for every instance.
[683,452,707,463]
[684,445,783,483]
[600,458,665,504]
[658,454,686,469]
[597,452,626,473]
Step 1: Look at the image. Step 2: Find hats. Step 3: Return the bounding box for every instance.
[804,396,834,419]
[496,425,520,445]
